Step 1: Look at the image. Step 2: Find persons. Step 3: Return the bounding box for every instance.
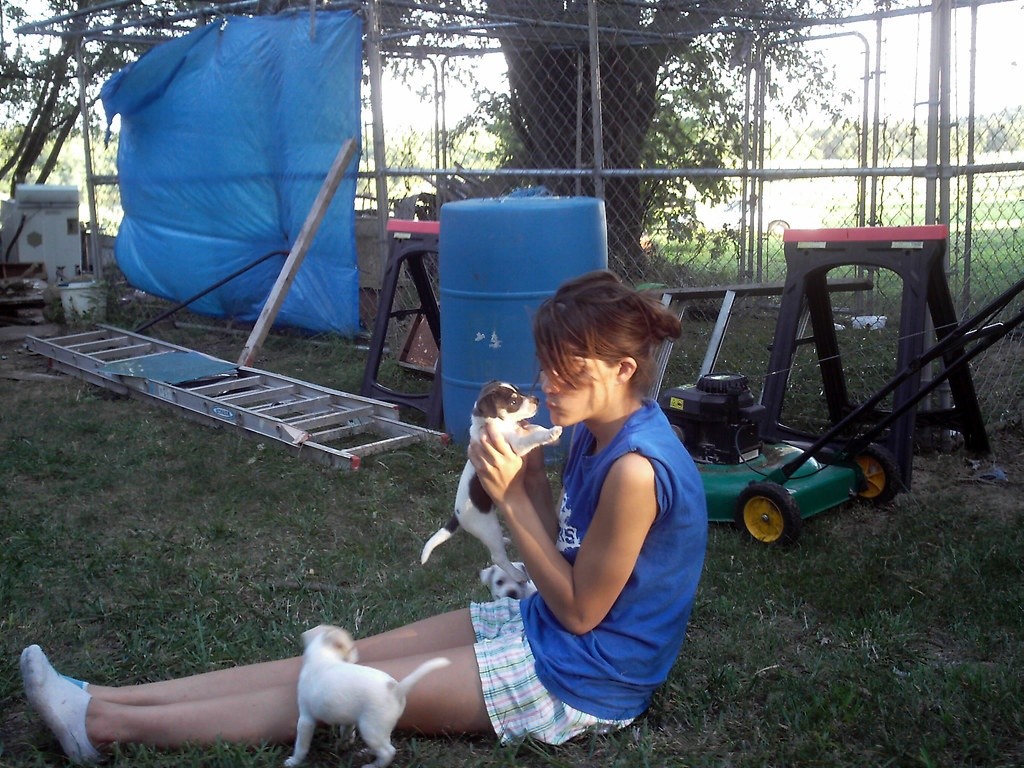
[19,269,708,766]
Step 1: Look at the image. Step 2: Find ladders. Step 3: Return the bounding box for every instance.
[396,275,874,409]
[26,321,453,469]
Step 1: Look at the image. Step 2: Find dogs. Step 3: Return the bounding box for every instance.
[479,561,538,601]
[419,379,563,585]
[283,624,452,768]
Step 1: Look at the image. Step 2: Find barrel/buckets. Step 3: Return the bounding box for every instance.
[441,197,606,463]
[58,281,108,326]
